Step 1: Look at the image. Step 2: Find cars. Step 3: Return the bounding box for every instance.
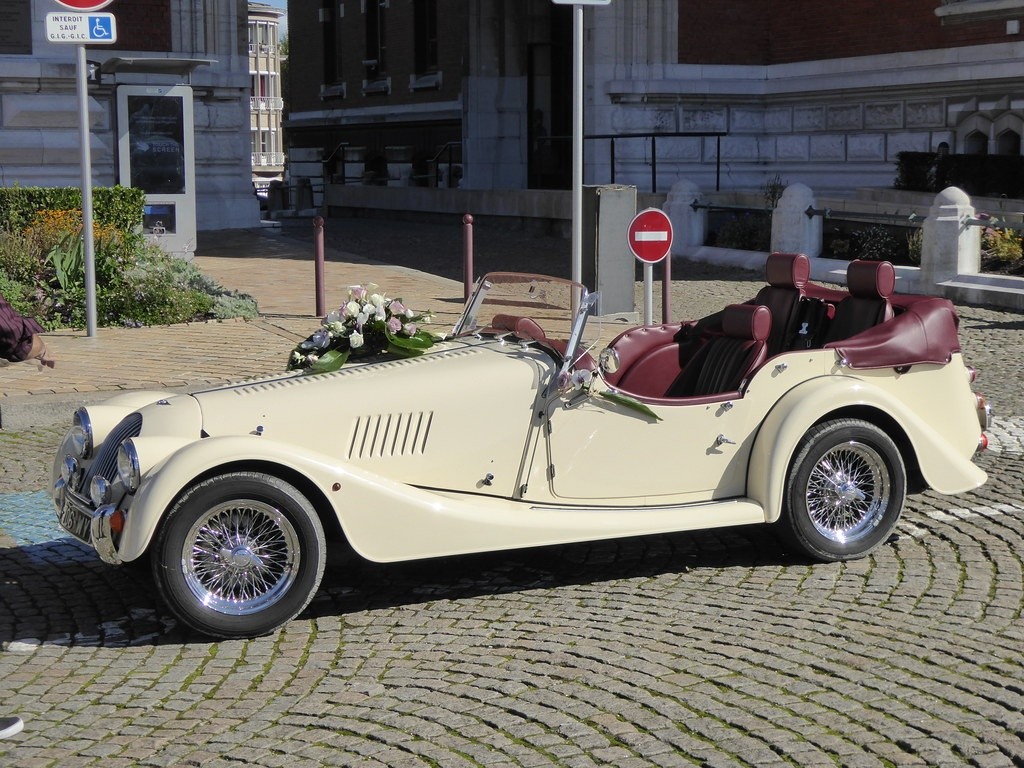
[47,254,988,641]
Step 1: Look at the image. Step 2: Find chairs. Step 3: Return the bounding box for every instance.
[691,304,772,395]
[493,314,606,383]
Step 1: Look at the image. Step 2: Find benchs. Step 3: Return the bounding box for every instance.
[754,252,895,358]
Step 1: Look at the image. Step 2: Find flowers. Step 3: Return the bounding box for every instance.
[286,282,437,372]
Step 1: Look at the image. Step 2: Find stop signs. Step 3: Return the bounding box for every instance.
[626,207,673,264]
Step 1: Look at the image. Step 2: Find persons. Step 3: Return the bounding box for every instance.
[0,294,54,429]
